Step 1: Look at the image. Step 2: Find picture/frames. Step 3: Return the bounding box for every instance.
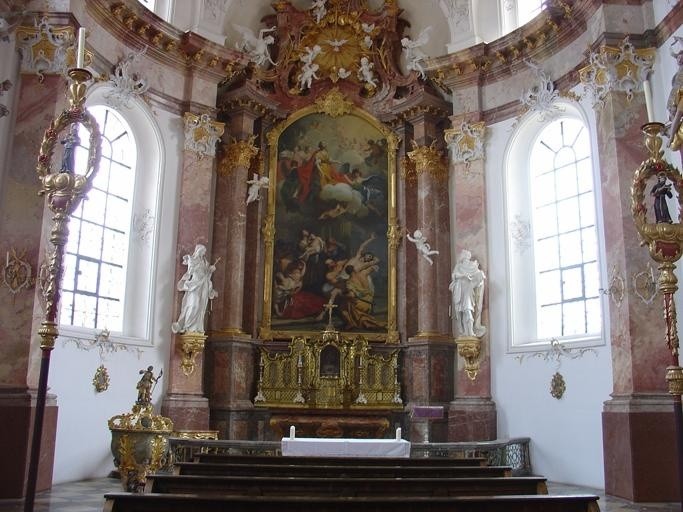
[256,85,404,346]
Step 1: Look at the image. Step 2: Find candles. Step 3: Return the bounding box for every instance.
[641,79,655,123]
[76,26,85,69]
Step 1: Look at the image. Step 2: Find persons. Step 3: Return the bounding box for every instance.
[450,248,484,337]
[133,364,157,402]
[252,25,281,69]
[648,172,675,226]
[399,36,429,81]
[272,140,385,332]
[241,172,271,208]
[295,44,379,93]
[310,0,328,25]
[662,49,682,150]
[405,227,440,266]
[56,123,79,176]
[173,243,218,333]
